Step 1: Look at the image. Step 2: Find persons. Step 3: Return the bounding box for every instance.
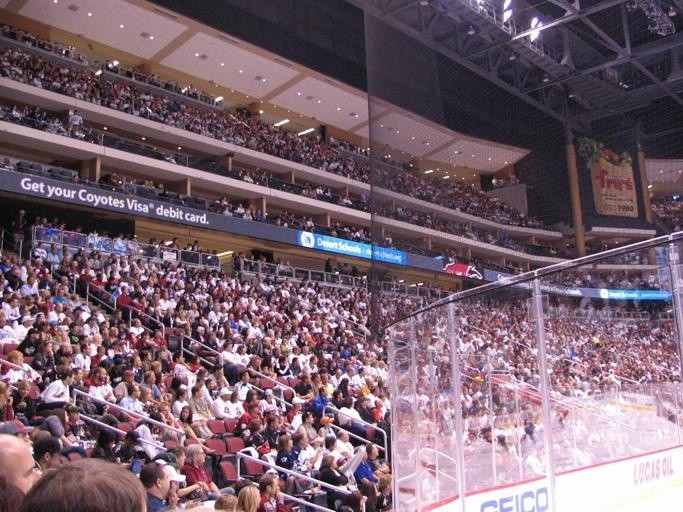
[0,23,683,511]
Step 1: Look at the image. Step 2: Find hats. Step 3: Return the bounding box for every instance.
[292,397,306,405]
[152,452,179,463]
[123,370,136,376]
[164,464,186,482]
[319,415,334,425]
[265,405,277,412]
[126,431,143,447]
[0,419,34,436]
[220,387,234,397]
[263,388,272,395]
